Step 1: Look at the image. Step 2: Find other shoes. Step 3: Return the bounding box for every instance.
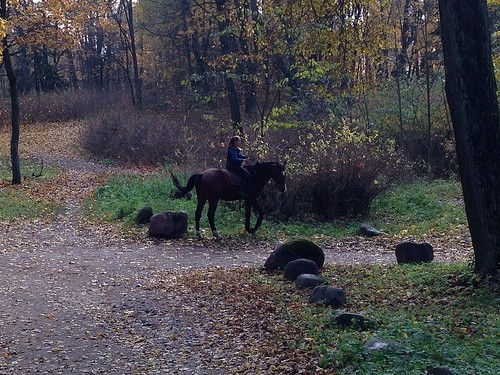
[237,191,247,197]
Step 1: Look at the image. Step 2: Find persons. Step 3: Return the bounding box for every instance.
[226,135,255,198]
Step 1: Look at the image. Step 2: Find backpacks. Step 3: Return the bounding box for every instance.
[226,150,232,171]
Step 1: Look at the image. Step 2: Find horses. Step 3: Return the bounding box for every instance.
[170,159,288,240]
